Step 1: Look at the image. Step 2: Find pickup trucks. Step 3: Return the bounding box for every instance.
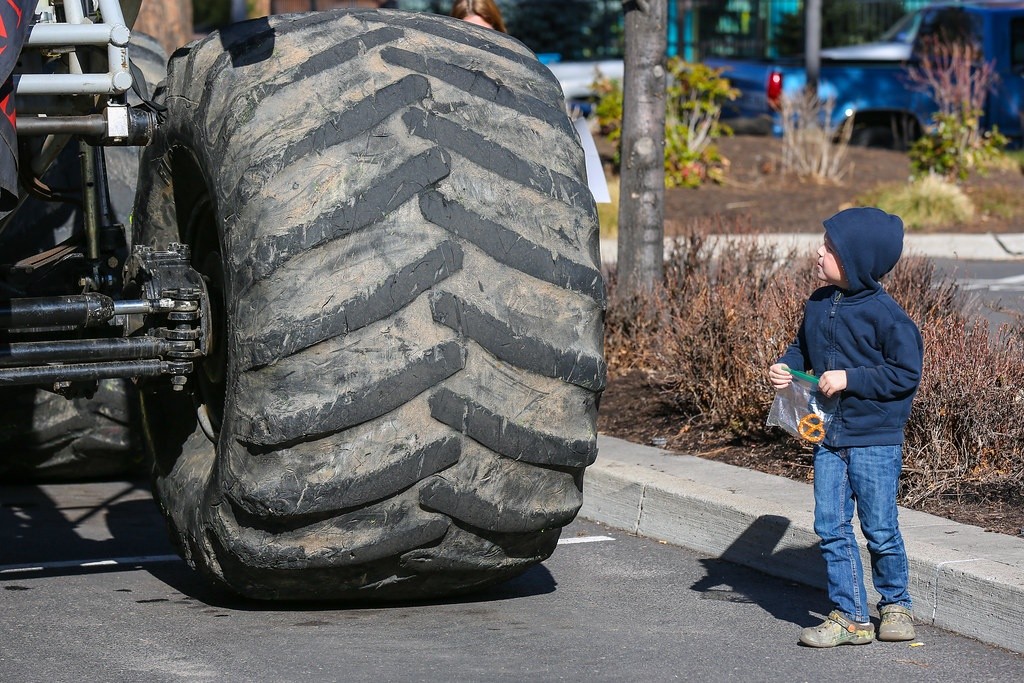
[678,0,1023,152]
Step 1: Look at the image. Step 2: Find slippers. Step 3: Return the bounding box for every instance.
[878,603,915,641]
[799,610,875,647]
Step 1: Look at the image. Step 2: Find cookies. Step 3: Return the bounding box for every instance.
[798,414,825,442]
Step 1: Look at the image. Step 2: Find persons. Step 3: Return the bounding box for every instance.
[769,206,923,649]
[452,0,507,33]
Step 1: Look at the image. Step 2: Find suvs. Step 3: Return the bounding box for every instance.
[0,0,606,605]
